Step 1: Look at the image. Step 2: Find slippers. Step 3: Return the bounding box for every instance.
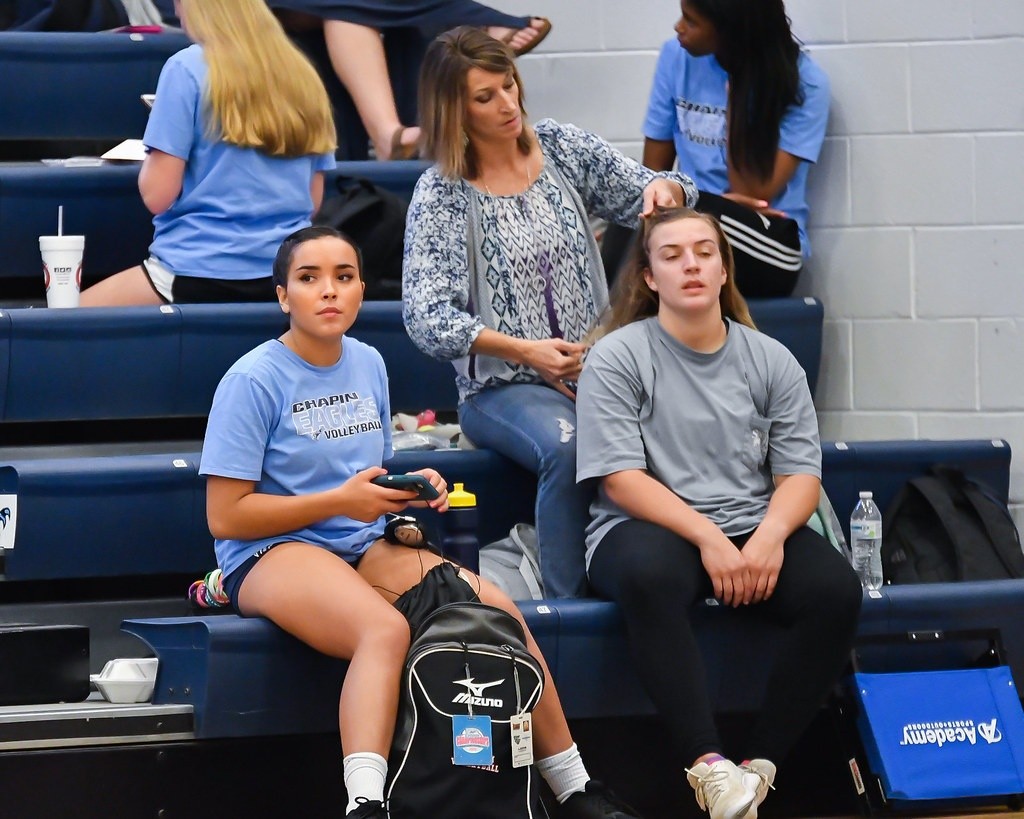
[481,16,551,58]
[390,126,421,162]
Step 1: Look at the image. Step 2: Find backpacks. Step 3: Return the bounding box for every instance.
[383,603,550,819]
[883,466,1024,585]
[312,176,408,300]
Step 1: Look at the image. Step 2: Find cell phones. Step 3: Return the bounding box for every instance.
[370,474,440,501]
[140,94,156,109]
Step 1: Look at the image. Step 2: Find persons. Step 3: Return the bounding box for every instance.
[576,204,862,819]
[198,227,641,818]
[403,26,699,599]
[601,0,829,287]
[82,0,336,307]
[264,1,550,159]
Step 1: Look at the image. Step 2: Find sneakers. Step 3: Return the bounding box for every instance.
[739,759,776,819]
[345,796,404,819]
[560,779,642,819]
[684,760,762,819]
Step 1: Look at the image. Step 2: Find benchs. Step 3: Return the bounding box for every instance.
[0,31,1023,819]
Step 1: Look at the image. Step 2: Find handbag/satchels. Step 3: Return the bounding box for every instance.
[478,523,550,605]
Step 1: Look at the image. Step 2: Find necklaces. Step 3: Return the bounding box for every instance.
[483,164,547,291]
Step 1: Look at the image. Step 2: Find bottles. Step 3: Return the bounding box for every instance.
[440,483,480,577]
[850,491,884,589]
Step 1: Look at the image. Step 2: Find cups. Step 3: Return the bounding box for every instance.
[39,235,85,308]
